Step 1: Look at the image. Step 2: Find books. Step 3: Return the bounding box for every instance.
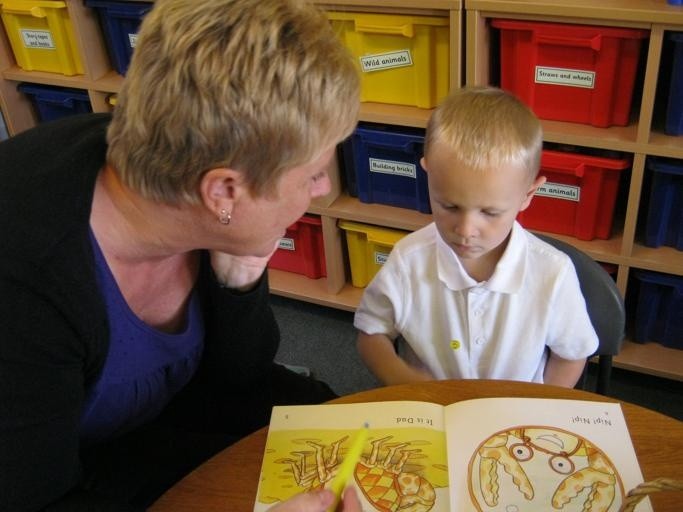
[252,395,654,512]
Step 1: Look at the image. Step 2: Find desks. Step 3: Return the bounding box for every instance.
[148,380,680,511]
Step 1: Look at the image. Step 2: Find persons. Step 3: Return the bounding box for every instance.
[1,3,371,511]
[351,85,600,391]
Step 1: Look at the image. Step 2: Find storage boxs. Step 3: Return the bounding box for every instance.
[629,269,681,351]
[267,216,327,280]
[645,160,681,251]
[320,11,451,109]
[660,31,682,137]
[342,129,434,215]
[491,19,644,129]
[1,1,86,77]
[16,82,93,126]
[82,2,154,78]
[516,149,628,241]
[338,220,411,288]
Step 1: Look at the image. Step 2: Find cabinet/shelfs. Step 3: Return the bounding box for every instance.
[0,1,682,379]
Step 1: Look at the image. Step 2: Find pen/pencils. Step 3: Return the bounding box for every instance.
[322,420,370,512]
